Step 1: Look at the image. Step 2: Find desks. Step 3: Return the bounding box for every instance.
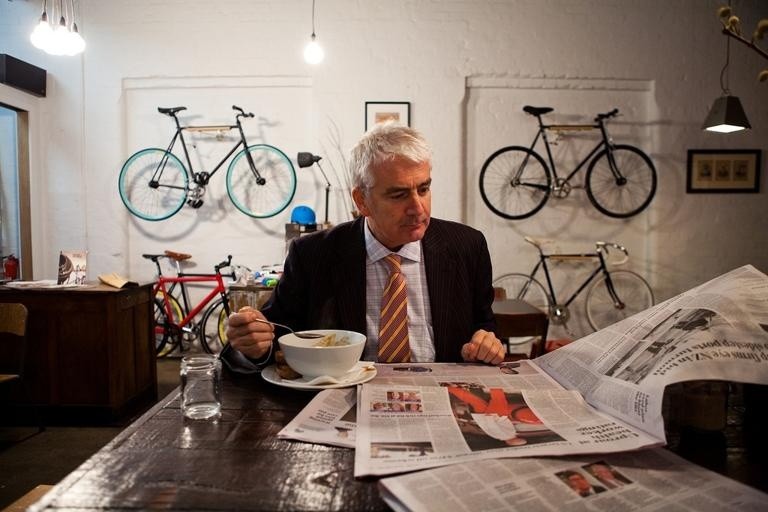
[0,280,160,426]
[491,298,549,359]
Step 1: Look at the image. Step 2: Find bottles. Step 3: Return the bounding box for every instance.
[180,354,221,421]
[6,255,17,279]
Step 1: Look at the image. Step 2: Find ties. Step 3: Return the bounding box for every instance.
[376,253,412,363]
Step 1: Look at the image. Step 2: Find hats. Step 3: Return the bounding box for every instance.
[291,205,318,226]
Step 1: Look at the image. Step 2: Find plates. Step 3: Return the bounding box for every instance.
[261,362,377,391]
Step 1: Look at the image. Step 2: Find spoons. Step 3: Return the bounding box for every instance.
[232,312,324,338]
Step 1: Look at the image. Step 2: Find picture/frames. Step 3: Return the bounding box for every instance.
[365,101,410,132]
[686,148,762,194]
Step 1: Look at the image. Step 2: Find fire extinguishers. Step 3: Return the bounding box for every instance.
[2,254,19,280]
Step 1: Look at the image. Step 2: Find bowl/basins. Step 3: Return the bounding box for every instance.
[278,330,366,381]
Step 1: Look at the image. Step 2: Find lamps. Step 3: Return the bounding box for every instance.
[304,0,325,67]
[30,1,86,58]
[701,35,751,133]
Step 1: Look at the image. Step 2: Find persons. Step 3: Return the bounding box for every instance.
[390,400,401,412]
[445,385,527,448]
[217,116,507,379]
[405,404,421,412]
[405,391,420,401]
[373,401,386,413]
[388,392,400,399]
[568,470,604,497]
[594,462,629,489]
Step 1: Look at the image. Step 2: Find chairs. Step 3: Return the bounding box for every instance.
[0,303,29,381]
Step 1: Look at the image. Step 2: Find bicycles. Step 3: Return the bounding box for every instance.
[477,103,662,224]
[141,252,233,361]
[114,103,299,222]
[150,247,230,357]
[487,235,659,339]
